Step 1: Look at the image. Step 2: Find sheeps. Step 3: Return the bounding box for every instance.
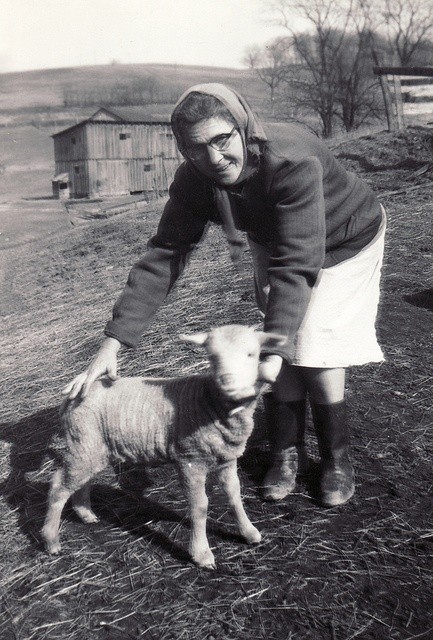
[39,324,289,572]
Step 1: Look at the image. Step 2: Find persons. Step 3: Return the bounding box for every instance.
[59,82,388,505]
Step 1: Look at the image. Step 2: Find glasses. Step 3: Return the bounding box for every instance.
[181,126,240,159]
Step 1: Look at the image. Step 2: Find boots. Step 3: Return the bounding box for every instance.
[259,393,306,501]
[313,397,357,507]
